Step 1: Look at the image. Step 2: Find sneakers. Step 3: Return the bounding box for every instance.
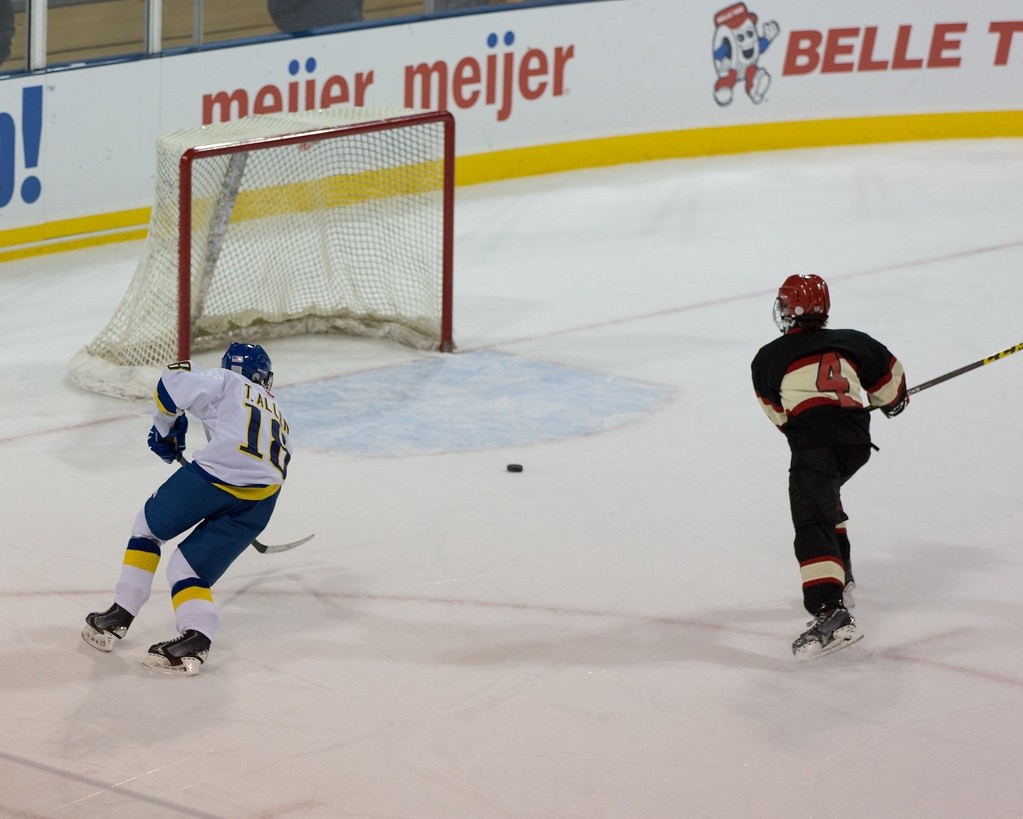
[792,600,863,661]
[841,567,856,608]
[140,628,211,678]
[81,603,136,652]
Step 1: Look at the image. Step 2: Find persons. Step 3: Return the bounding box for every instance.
[268,0,364,33]
[82,342,290,675]
[0,0,16,68]
[751,273,910,661]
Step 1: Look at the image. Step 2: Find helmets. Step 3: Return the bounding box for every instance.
[774,275,830,333]
[221,342,272,390]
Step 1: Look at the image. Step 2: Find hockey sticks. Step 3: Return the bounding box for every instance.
[863,342,1023,413]
[159,437,317,556]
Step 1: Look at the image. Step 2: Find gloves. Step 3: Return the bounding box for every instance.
[886,394,911,416]
[147,413,187,465]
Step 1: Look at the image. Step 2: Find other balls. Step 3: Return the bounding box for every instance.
[506,463,523,472]
